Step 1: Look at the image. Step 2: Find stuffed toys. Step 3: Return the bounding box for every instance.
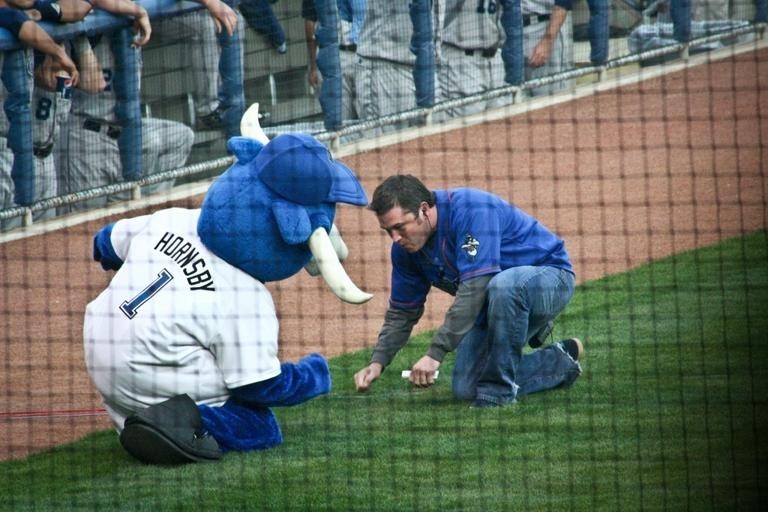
[81,101,374,466]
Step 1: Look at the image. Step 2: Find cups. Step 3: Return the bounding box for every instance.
[56,76,73,102]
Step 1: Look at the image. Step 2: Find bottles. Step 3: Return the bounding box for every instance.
[141,103,151,118]
[181,92,196,131]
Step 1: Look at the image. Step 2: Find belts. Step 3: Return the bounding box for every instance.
[31,137,55,159]
[341,45,356,53]
[441,42,500,53]
[523,14,550,26]
[83,120,120,141]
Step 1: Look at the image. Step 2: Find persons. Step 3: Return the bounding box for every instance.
[0,0,575,231]
[604,0,757,38]
[352,172,583,408]
